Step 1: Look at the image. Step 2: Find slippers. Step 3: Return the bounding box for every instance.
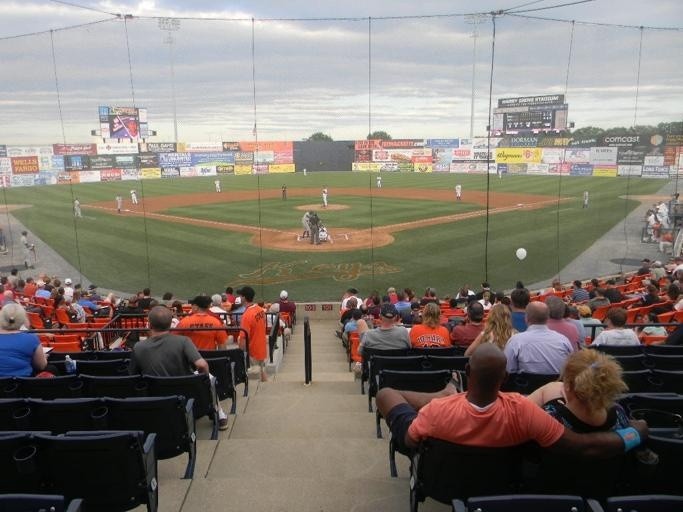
[449,370,464,394]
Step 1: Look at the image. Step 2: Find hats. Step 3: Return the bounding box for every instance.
[577,305,592,316]
[280,290,288,299]
[380,302,396,319]
[640,259,649,262]
[237,287,254,297]
[65,278,72,284]
[481,282,491,291]
[89,284,98,290]
[188,296,212,305]
[411,302,420,311]
[80,291,92,297]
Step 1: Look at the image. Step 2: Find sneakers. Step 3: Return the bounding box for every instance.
[218,413,229,429]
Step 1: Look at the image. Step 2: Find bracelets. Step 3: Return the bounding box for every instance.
[615,426,641,452]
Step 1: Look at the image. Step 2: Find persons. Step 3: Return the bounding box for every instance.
[377,174,382,189]
[523,347,630,434]
[215,177,221,193]
[320,184,328,208]
[1,268,296,429]
[498,168,502,184]
[130,187,139,205]
[19,230,37,271]
[375,343,650,463]
[454,185,464,200]
[114,195,124,214]
[335,260,682,407]
[645,192,683,251]
[582,189,589,208]
[281,183,288,200]
[73,197,84,218]
[301,209,328,245]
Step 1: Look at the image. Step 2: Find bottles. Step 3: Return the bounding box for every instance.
[64,354,74,374]
